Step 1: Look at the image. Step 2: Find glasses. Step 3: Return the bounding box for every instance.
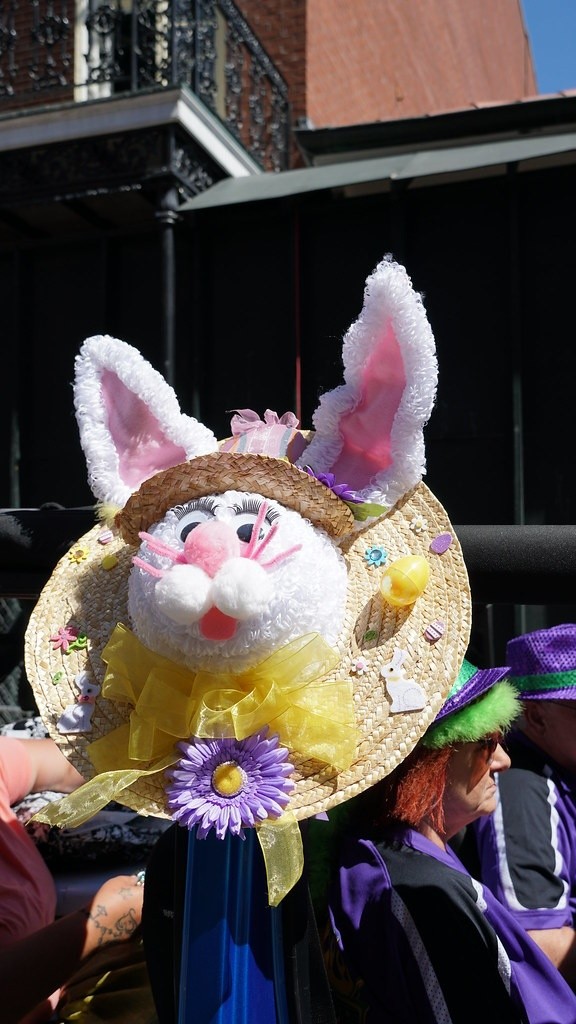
[466,734,497,763]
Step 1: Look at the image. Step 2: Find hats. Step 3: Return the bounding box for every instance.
[26,260,473,841]
[416,660,522,746]
[507,622,576,703]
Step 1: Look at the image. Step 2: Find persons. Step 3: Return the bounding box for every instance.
[0,623,575,1023]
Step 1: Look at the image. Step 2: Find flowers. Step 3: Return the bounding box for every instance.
[163,726,296,843]
